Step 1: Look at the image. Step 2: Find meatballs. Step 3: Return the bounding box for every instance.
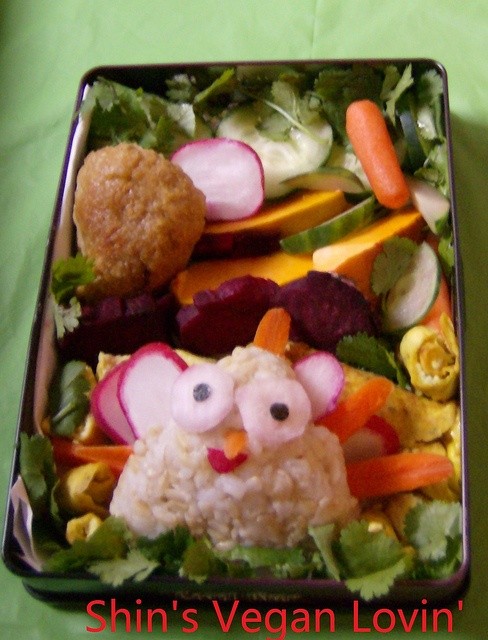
[72,142,207,294]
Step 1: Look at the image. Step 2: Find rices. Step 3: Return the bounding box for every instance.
[108,343,363,549]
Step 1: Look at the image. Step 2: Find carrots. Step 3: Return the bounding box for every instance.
[346,99,411,210]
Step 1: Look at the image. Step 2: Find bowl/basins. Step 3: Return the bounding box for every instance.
[2,59,473,607]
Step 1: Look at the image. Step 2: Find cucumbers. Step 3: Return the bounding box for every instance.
[320,165,367,197]
[403,172,452,234]
[280,195,375,256]
[218,102,333,198]
[381,241,442,337]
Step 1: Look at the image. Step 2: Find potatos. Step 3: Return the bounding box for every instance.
[173,272,375,351]
[59,295,175,363]
